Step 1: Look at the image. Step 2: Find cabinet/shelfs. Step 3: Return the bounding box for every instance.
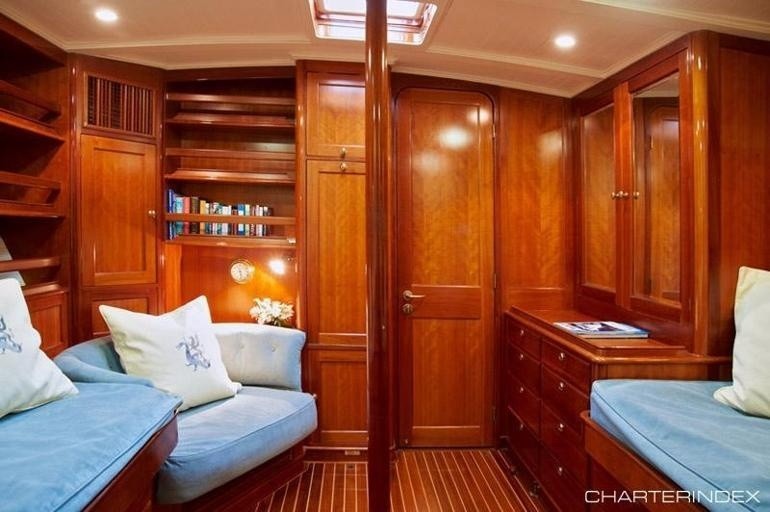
[505,312,732,512]
[298,62,367,353]
[0,15,292,353]
[571,31,769,351]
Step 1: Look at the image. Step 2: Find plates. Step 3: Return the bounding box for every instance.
[298,62,367,353]
[571,31,769,351]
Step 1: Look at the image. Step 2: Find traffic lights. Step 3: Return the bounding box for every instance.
[0,277,78,420]
[97,292,240,413]
[710,264,770,419]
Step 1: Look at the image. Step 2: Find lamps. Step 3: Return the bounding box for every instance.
[54,318,319,512]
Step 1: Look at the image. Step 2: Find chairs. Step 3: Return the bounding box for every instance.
[54,318,319,512]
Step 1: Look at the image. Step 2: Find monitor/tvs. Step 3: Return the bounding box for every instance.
[0,238,26,287]
[553,320,650,339]
[168,189,273,240]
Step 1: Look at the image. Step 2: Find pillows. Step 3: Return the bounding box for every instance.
[0,277,78,420]
[97,292,240,413]
[710,264,770,419]
